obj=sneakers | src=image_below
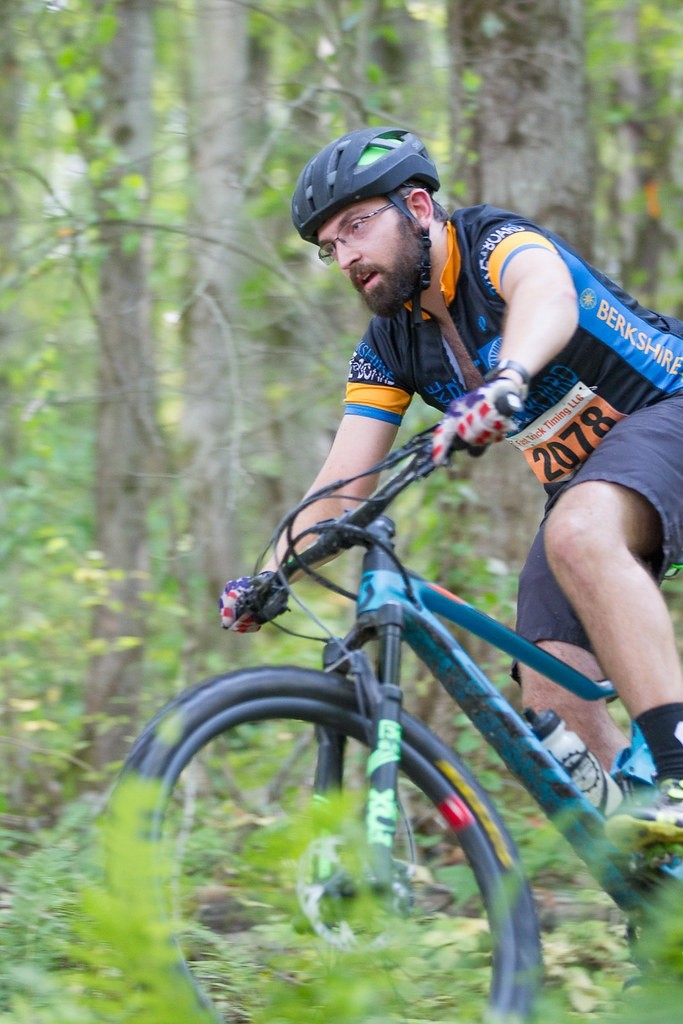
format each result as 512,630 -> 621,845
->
605,778 -> 683,854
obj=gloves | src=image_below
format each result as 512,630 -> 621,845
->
431,378 -> 521,464
218,571 -> 275,632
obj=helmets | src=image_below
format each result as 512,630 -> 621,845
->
291,126 -> 440,245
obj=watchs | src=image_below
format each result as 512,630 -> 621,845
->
485,359 -> 529,386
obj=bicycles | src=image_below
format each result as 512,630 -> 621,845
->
111,424 -> 682,1024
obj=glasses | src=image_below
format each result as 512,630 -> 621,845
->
318,187 -> 428,265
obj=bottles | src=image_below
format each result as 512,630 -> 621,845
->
522,707 -> 623,815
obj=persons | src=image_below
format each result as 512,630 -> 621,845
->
219,121 -> 683,887
